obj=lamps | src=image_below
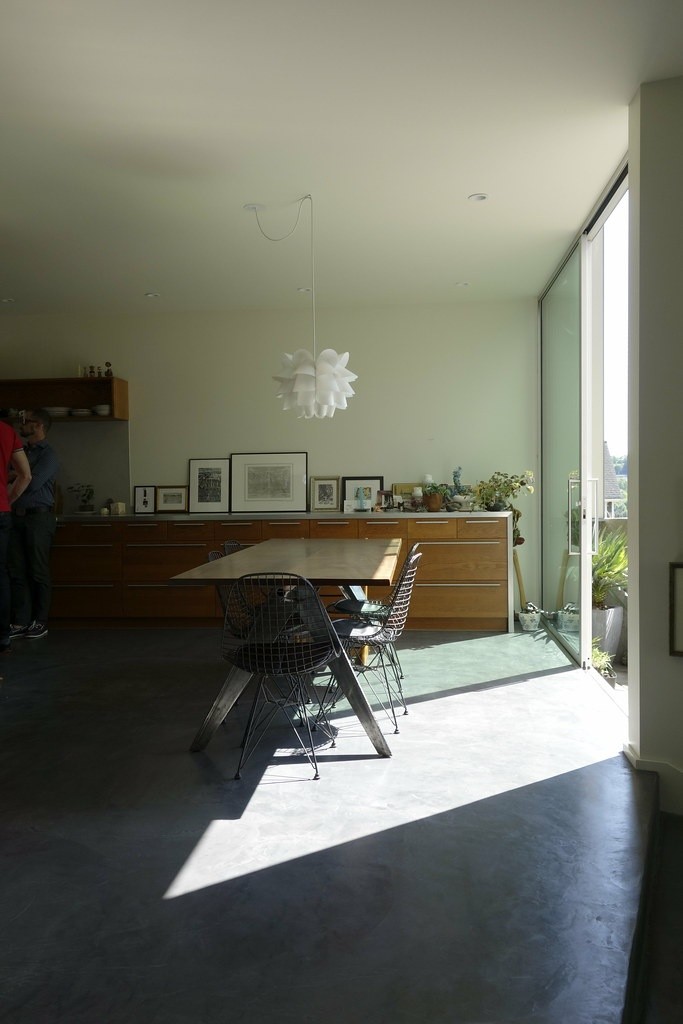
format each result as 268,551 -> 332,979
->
243,197 -> 359,419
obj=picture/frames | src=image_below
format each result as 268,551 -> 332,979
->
310,476 -> 341,512
188,458 -> 230,513
341,476 -> 384,511
155,485 -> 188,513
230,452 -> 308,512
134,486 -> 155,513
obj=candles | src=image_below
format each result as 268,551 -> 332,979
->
414,487 -> 423,496
424,474 -> 432,483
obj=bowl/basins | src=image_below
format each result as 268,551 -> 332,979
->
95,405 -> 111,416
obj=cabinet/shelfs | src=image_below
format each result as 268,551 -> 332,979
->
49,514 -> 514,633
0,377 -> 129,421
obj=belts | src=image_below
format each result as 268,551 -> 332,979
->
12,504 -> 53,517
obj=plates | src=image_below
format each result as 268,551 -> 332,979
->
40,407 -> 71,417
70,409 -> 93,416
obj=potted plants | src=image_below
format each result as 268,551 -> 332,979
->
558,602 -> 580,631
592,525 -> 628,666
423,465 -> 534,546
68,483 -> 95,512
592,636 -> 617,689
518,601 -> 541,631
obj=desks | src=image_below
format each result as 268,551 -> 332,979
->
167,538 -> 402,757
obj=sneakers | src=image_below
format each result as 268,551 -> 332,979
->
6,624 -> 30,639
25,620 -> 49,638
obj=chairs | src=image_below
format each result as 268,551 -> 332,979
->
203,539 -> 422,780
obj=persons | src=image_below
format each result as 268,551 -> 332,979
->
0,417 -> 32,651
23,410 -> 63,640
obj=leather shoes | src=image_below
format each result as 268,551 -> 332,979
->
0,637 -> 13,652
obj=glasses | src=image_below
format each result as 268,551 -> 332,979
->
21,419 -> 39,425
